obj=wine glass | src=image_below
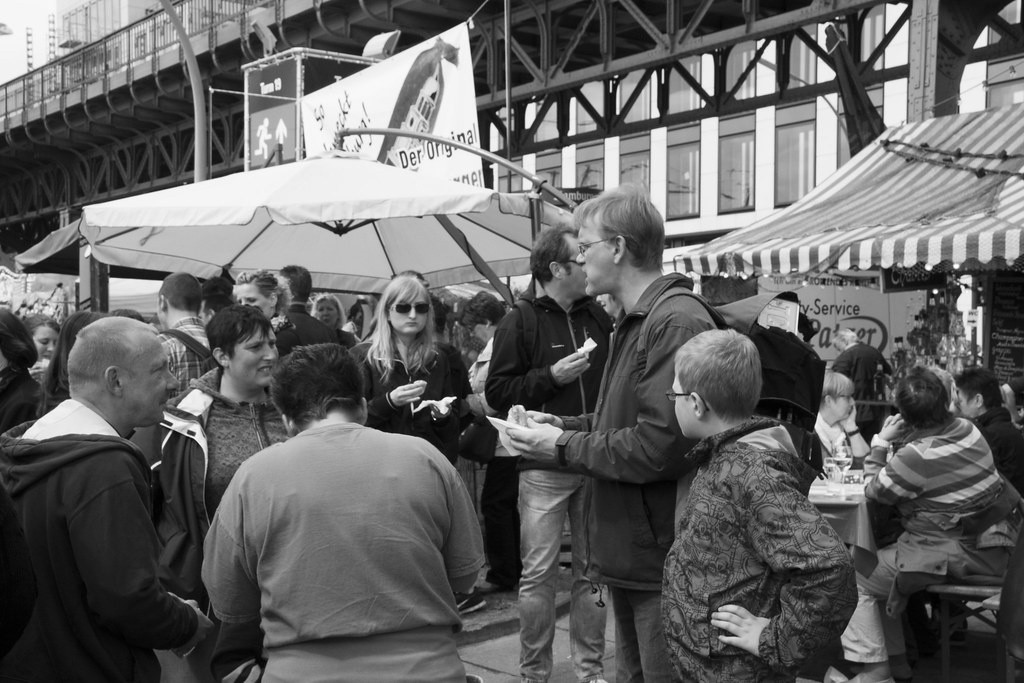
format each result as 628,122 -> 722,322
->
824,445 -> 854,494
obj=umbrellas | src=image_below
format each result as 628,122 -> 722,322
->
13,125 -> 589,299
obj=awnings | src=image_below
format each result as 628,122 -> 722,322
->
672,102 -> 1024,280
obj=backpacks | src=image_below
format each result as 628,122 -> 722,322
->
635,289 -> 823,470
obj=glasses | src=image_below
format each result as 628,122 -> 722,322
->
578,237 -> 612,256
390,303 -> 429,313
839,394 -> 851,399
467,324 -> 477,332
665,389 -> 710,412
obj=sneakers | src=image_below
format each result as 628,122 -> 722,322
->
453,586 -> 486,615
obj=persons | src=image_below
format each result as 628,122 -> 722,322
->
201,344 -> 489,681
0,264 -> 1023,683
658,328 -> 858,683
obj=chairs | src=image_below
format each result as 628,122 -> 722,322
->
923,583 -> 1024,683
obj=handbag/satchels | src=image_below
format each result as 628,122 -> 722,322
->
458,416 -> 499,466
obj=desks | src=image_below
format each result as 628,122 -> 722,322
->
809,469 -> 898,579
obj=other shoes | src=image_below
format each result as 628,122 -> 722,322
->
906,652 -> 919,666
847,664 -> 914,683
586,679 -> 609,683
929,614 -> 969,645
486,570 -> 513,592
915,645 -> 935,656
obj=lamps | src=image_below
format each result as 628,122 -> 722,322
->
361,29 -> 402,59
